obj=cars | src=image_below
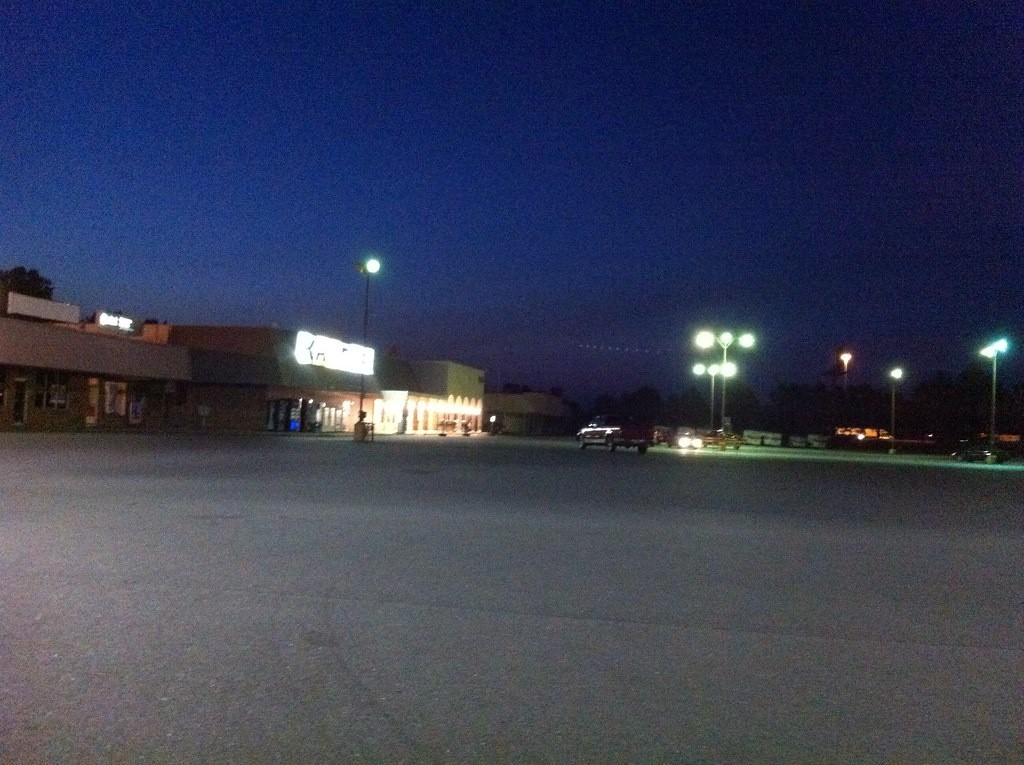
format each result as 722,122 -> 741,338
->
703,430 -> 744,449
666,427 -> 703,449
947,442 -> 1010,464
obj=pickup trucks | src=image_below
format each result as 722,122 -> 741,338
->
576,413 -> 655,454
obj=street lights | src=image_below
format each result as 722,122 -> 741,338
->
490,415 -> 496,432
888,368 -> 902,455
840,353 -> 852,384
980,338 -> 1008,442
359,259 -> 381,421
693,331 -> 755,430
